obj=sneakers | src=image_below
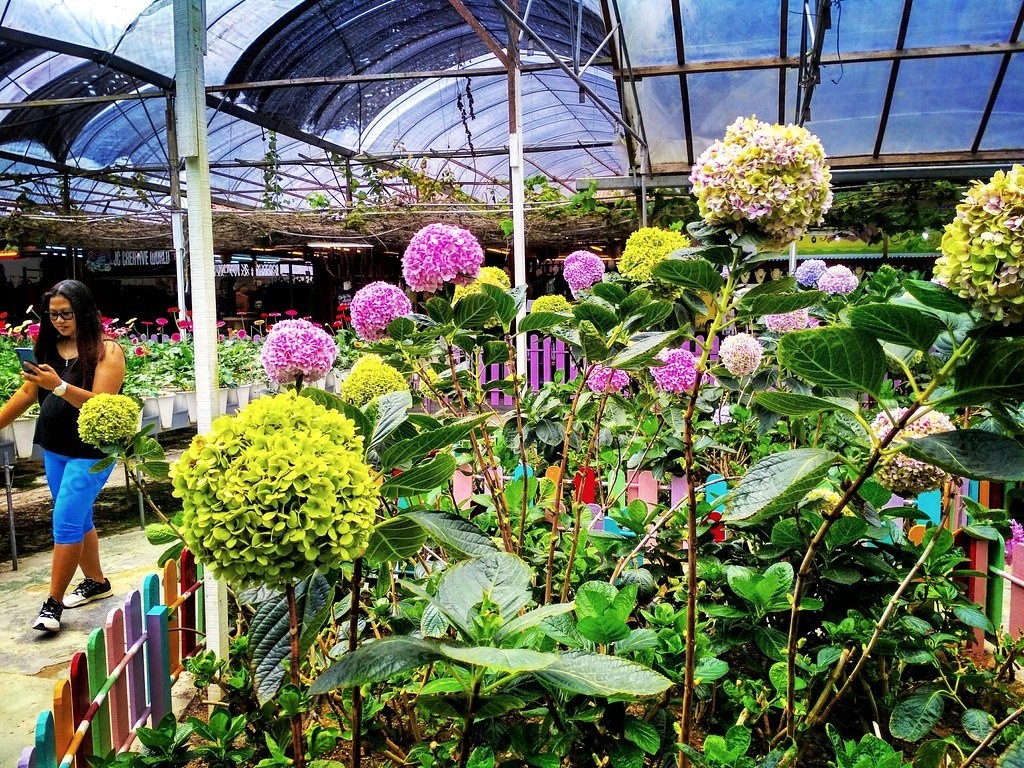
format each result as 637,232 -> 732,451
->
31,596 -> 66,632
62,577 -> 114,608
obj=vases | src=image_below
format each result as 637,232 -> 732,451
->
250,382 -> 271,399
327,368 -> 341,388
311,376 -> 326,390
0,418 -> 37,458
143,392 -> 176,428
185,389 -> 229,421
168,389 -> 188,415
0,303 -> 360,418
335,371 -> 350,393
228,383 -> 252,411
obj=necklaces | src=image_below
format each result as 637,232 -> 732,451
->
64,341 -> 77,366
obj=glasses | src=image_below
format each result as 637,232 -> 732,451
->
44,311 -> 74,321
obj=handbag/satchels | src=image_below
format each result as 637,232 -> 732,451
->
42,356 -> 94,424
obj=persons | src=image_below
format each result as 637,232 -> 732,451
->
0,280 -> 126,630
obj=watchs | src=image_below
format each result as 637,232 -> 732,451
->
52,380 -> 69,396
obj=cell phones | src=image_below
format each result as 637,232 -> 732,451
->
14,348 -> 39,375
